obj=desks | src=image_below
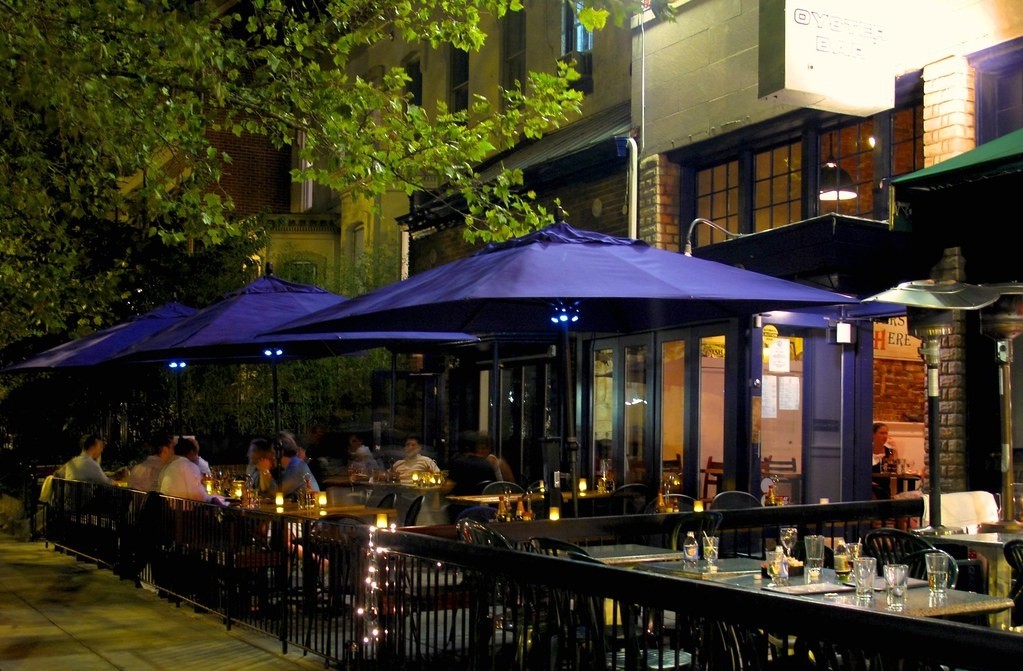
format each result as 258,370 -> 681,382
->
871,471 -> 920,480
922,531 -> 1023,635
222,475 -> 451,619
793,589 -> 1014,671
713,572 -> 930,597
445,492 -> 544,507
575,543 -> 683,566
560,489 -> 610,499
636,557 -> 772,580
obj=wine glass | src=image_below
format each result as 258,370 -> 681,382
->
780,528 -> 798,557
702,537 -> 719,570
766,551 -> 784,587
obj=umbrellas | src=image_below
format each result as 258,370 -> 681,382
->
82,262 -> 482,492
255,203 -> 864,516
0,300 -> 289,439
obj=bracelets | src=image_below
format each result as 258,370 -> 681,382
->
263,470 -> 270,475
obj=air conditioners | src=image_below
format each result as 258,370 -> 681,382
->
556,49 -> 591,79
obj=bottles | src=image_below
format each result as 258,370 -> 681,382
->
495,496 -> 533,522
764,485 -> 789,506
654,489 -> 680,513
883,457 -> 915,475
296,485 -> 319,511
683,531 -> 699,571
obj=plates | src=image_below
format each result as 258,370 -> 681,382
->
760,583 -> 856,595
843,576 -> 929,591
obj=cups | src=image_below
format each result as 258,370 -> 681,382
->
883,564 -> 909,612
834,540 -> 863,584
925,553 -> 949,593
804,535 -> 825,568
853,557 -> 877,601
411,469 -> 444,487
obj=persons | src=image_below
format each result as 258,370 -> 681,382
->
381,432 -> 441,478
872,422 -> 900,473
245,430 -> 320,539
306,425 -> 372,466
54,434 -> 120,512
440,432 -> 515,513
127,435 -> 213,543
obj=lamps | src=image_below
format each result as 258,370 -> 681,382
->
818,131 -> 858,200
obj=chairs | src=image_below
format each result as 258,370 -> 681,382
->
405,494 -> 424,528
452,455 -> 1023,671
376,492 -> 398,508
290,514 -> 369,631
